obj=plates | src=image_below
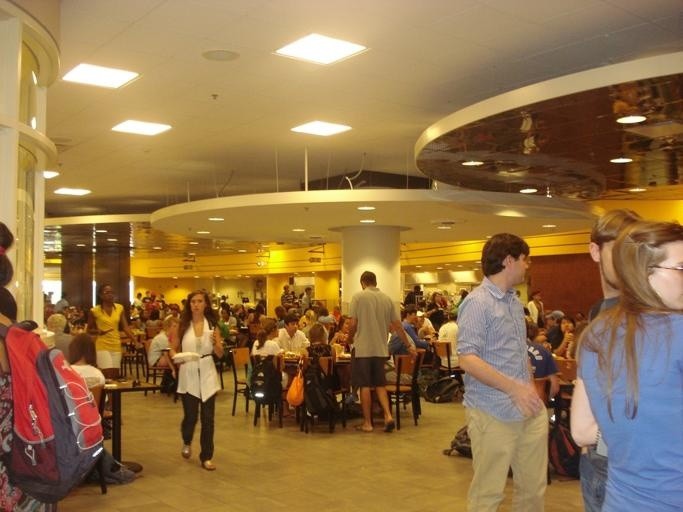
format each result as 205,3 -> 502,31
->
283,345 -> 352,362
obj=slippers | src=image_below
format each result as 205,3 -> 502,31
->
354,424 -> 373,432
384,418 -> 395,432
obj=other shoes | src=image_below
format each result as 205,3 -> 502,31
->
182,444 -> 192,460
203,461 -> 216,471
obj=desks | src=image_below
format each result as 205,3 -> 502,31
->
215,344 -> 237,389
104,377 -> 162,475
281,352 -> 354,427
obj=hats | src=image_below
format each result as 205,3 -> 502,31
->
545,310 -> 565,320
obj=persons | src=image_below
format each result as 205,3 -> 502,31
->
387,283 -> 470,385
516,288 -> 587,398
455,232 -> 549,512
344,271 -> 412,433
0,312 -> 58,512
44,283 -> 186,408
216,284 -> 351,421
579,208 -> 642,512
168,290 -> 225,471
570,220 -> 682,512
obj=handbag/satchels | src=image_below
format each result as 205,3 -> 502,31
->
285,358 -> 306,407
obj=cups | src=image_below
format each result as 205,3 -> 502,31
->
207,329 -> 214,342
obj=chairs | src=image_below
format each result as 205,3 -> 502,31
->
249,355 -> 287,427
247,323 -> 259,348
229,347 -> 251,416
373,353 -> 422,431
120,334 -> 146,379
433,342 -> 465,389
142,339 -> 171,395
535,377 -> 552,408
415,348 -> 426,385
327,363 -> 352,427
296,358 -> 335,433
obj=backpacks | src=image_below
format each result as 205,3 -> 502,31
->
544,423 -> 577,480
304,356 -> 345,425
251,356 -> 281,407
443,423 -> 472,459
4,320 -> 104,501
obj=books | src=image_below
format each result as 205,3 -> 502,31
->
594,431 -> 609,458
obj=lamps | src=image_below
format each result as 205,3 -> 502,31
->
255,241 -> 270,268
308,239 -> 326,263
182,245 -> 196,270
